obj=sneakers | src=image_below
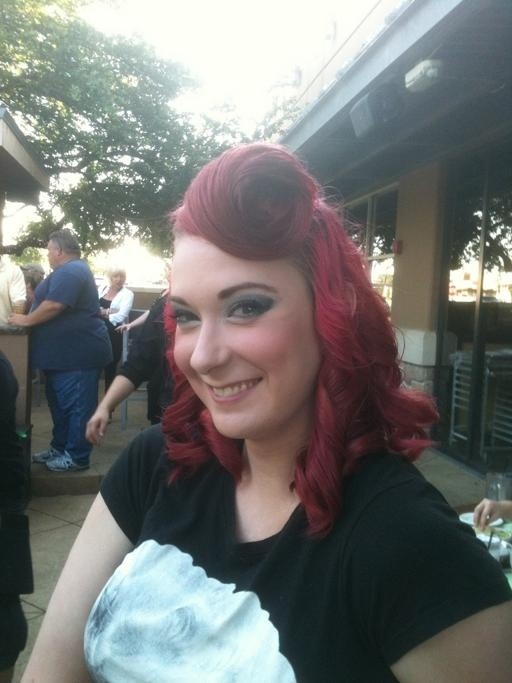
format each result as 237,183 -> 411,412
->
109,412 -> 113,424
32,447 -> 89,472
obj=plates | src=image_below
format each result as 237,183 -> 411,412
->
458,510 -> 504,528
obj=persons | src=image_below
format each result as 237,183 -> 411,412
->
0,228 -> 174,471
472,497 -> 512,531
23,142 -> 512,683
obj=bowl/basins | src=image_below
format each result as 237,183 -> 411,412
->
476,535 -> 500,549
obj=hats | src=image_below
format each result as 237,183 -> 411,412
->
20,262 -> 45,274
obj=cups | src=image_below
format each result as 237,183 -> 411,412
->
485,471 -> 506,501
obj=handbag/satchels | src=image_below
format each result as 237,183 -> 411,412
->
10,513 -> 35,596
